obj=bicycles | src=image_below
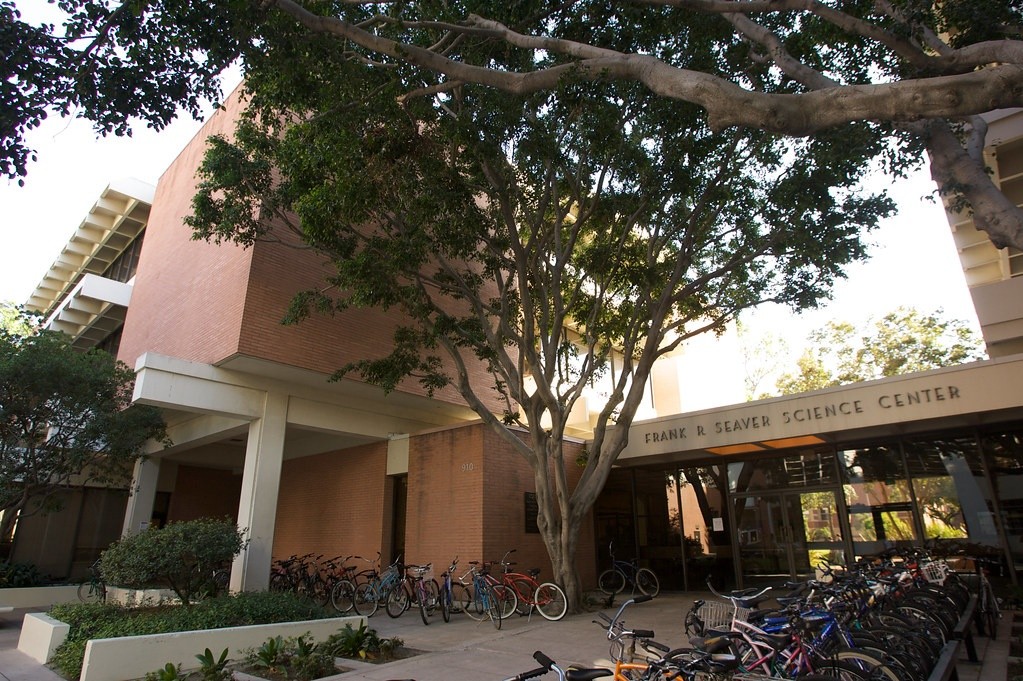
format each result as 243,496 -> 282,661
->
268,535 -> 1007,681
597,541 -> 661,601
77,558 -> 107,606
